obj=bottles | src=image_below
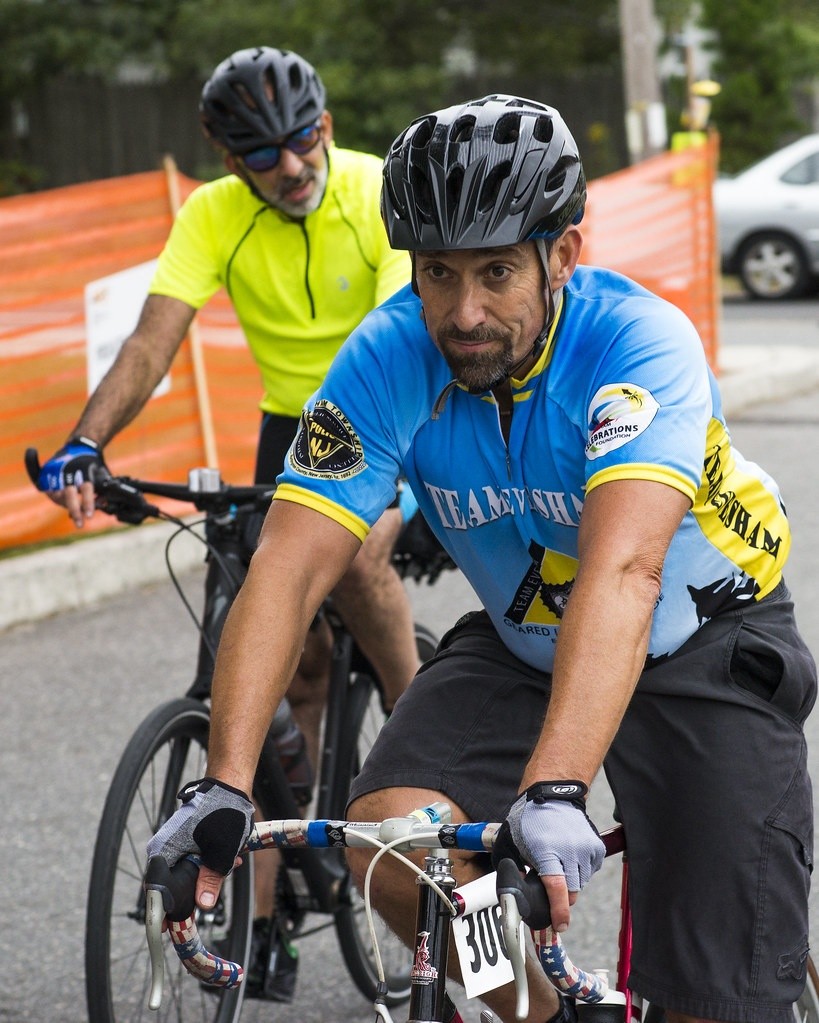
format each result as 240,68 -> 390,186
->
271,697 -> 314,805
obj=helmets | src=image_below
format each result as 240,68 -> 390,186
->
381,94 -> 586,252
199,45 -> 325,152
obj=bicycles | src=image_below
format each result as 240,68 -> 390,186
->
143,803 -> 819,1023
24,446 -> 442,1023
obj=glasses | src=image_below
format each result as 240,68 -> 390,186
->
238,120 -> 321,173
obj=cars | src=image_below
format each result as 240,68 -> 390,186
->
711,131 -> 819,301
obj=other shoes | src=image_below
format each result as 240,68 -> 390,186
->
201,937 -> 298,1002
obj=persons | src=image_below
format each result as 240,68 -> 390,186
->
145,94 -> 819,1023
37,46 -> 423,996
